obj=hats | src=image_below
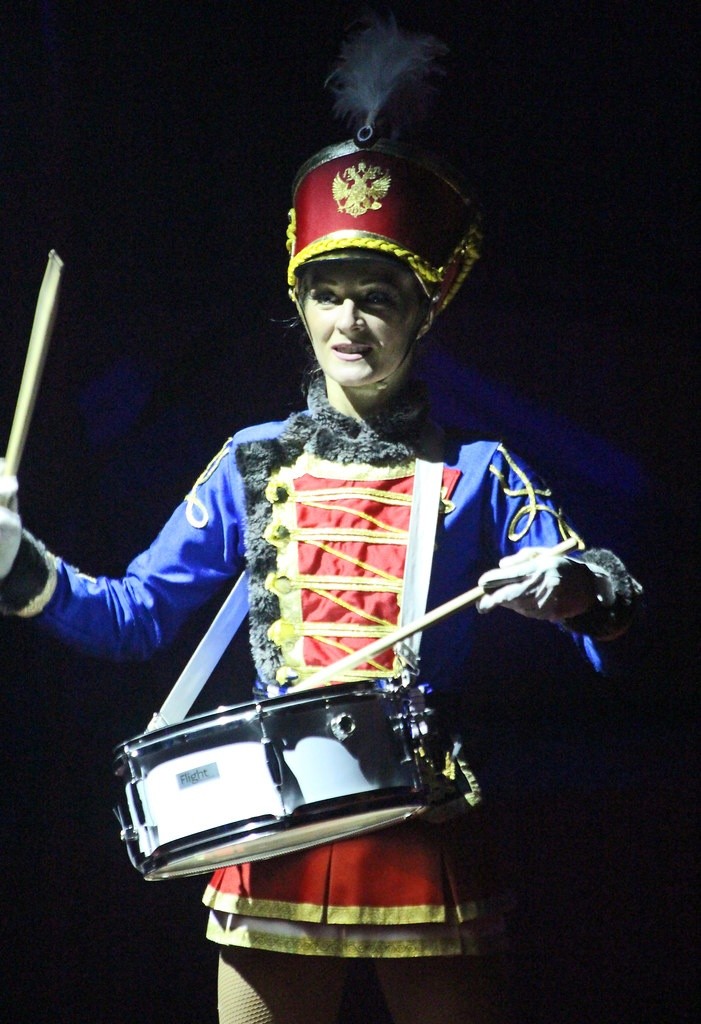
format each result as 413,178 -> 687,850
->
288,140 -> 485,316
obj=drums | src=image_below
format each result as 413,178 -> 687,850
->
104,673 -> 442,889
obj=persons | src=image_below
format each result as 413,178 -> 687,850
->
0,137 -> 672,1023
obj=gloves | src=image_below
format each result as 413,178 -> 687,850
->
475,546 -> 589,620
0,457 -> 22,580
